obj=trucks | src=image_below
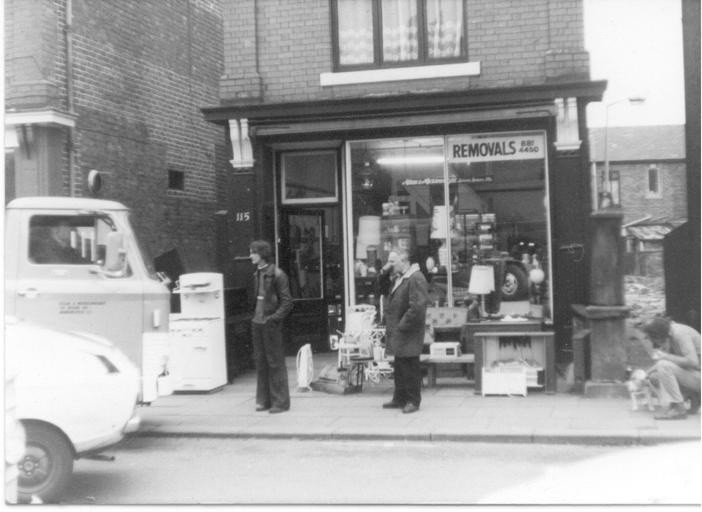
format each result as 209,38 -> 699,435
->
452,226 -> 527,299
5,173 -> 175,429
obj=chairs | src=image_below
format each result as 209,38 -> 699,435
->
337,304 -> 376,370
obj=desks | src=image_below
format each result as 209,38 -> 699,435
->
463,314 -> 543,380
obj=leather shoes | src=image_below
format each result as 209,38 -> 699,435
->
382,400 -> 419,414
256,403 -> 288,414
654,408 -> 687,421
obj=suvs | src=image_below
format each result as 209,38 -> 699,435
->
6,315 -> 146,505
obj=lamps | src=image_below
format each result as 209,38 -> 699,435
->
358,215 -> 381,267
429,205 -> 457,267
467,264 -> 501,318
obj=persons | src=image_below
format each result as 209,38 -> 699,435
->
246,241 -> 293,414
382,248 -> 428,413
31,216 -> 93,264
642,316 -> 701,419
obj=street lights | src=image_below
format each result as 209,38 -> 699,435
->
599,94 -> 648,195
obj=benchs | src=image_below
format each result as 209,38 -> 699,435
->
387,353 -> 476,388
